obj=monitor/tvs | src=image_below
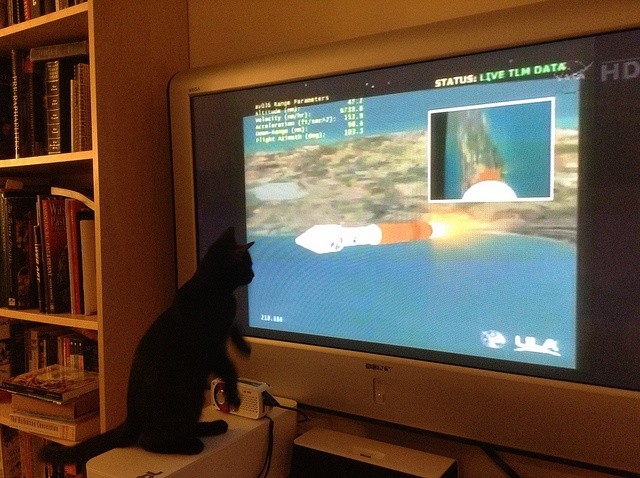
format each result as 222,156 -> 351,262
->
167,0 -> 640,477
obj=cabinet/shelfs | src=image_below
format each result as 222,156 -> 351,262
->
0,1 -> 188,478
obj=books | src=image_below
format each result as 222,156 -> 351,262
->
0,177 -> 97,317
11,41 -> 92,159
0,1 -> 87,28
0,323 -> 101,441
0,442 -> 87,478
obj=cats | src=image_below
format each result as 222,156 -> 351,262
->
38,225 -> 255,466
